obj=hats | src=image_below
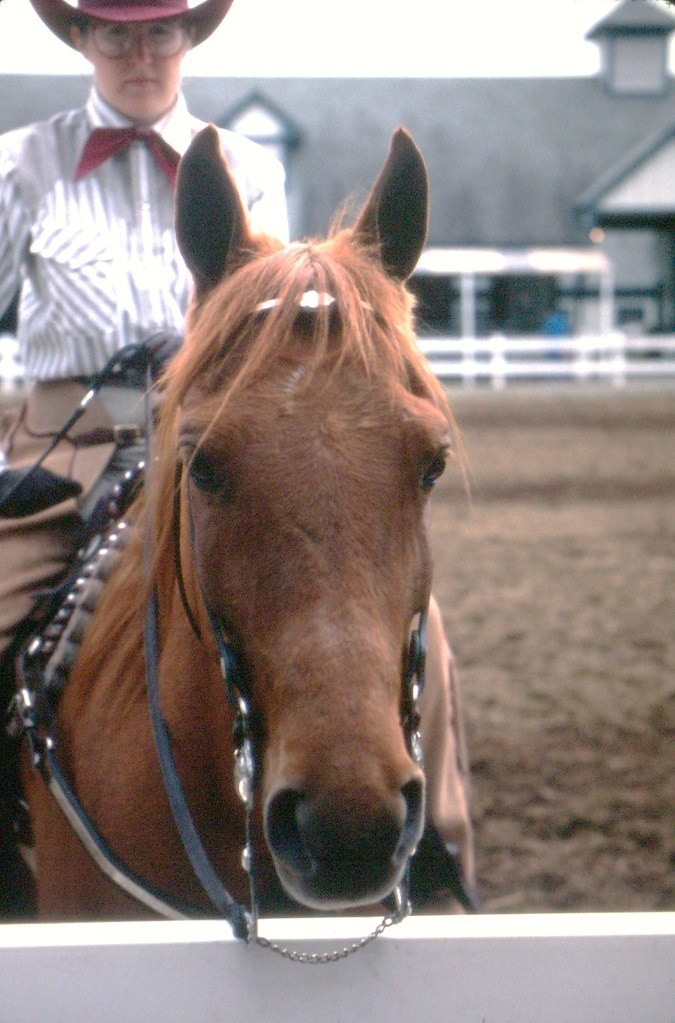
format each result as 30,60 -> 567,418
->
33,0 -> 233,53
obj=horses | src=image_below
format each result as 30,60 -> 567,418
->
26,122 -> 478,922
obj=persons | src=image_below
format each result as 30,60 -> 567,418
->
0,0 -> 474,916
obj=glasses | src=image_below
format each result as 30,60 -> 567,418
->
82,19 -> 188,59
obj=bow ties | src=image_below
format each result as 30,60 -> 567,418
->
73,126 -> 180,184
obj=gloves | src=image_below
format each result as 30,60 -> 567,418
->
0,465 -> 82,516
121,333 -> 184,374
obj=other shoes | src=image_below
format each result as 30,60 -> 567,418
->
415,892 -> 464,915
